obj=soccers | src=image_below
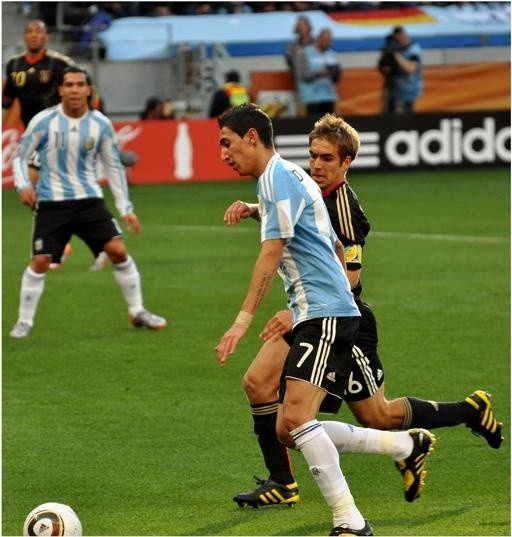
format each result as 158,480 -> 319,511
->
24,502 -> 81,536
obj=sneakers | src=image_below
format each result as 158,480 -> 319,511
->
232,474 -> 300,509
89,252 -> 109,272
9,321 -> 33,339
465,390 -> 503,449
395,429 -> 437,502
329,519 -> 375,537
48,244 -> 71,270
127,308 -> 167,331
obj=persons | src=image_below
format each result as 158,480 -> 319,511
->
207,70 -> 254,118
213,103 -> 436,534
139,97 -> 175,120
294,30 -> 343,132
379,25 -> 424,111
223,113 -> 504,508
3,20 -> 138,170
285,15 -> 322,70
8,67 -> 167,338
